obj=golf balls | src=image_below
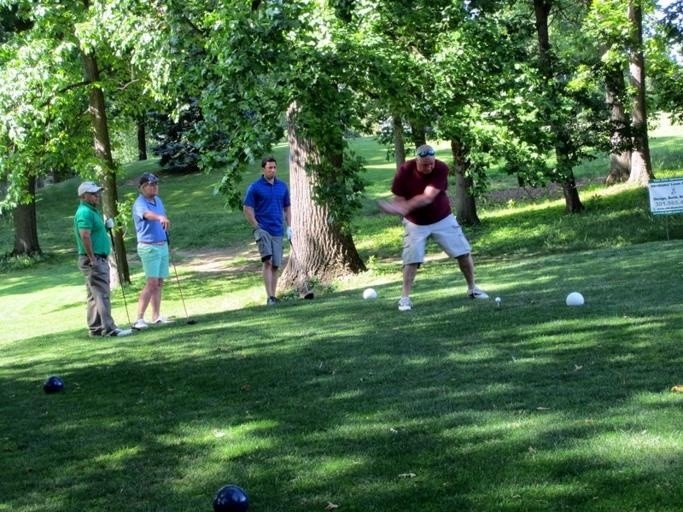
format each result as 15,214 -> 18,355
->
362,288 -> 377,301
495,298 -> 502,304
566,292 -> 584,307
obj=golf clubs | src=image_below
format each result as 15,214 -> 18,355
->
288,238 -> 313,297
109,225 -> 138,329
164,228 -> 189,320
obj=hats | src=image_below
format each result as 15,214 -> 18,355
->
140,173 -> 159,184
78,182 -> 104,196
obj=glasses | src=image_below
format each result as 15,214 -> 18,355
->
418,150 -> 434,157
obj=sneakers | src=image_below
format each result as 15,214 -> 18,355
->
88,319 -> 173,337
268,296 -> 276,305
468,287 -> 489,299
397,297 -> 412,311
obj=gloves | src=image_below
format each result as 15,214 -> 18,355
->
105,218 -> 114,228
286,226 -> 291,239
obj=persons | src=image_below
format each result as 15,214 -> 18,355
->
129,172 -> 175,330
71,182 -> 132,337
376,144 -> 489,311
243,156 -> 292,306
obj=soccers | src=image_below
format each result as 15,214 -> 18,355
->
43,376 -> 65,394
212,485 -> 248,511
